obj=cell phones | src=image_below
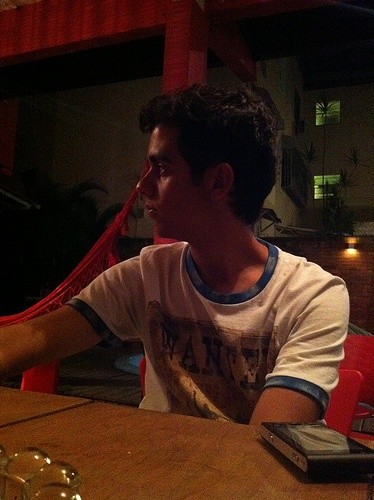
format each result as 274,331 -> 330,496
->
260,420 -> 374,477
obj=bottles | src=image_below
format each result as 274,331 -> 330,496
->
4,448 -> 49,500
27,461 -> 82,500
0,445 -> 11,473
1,473 -> 28,500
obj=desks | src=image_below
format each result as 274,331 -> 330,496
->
0,387 -> 374,499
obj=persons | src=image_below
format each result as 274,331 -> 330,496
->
0,83 -> 351,427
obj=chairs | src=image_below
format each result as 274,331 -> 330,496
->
138,332 -> 374,441
